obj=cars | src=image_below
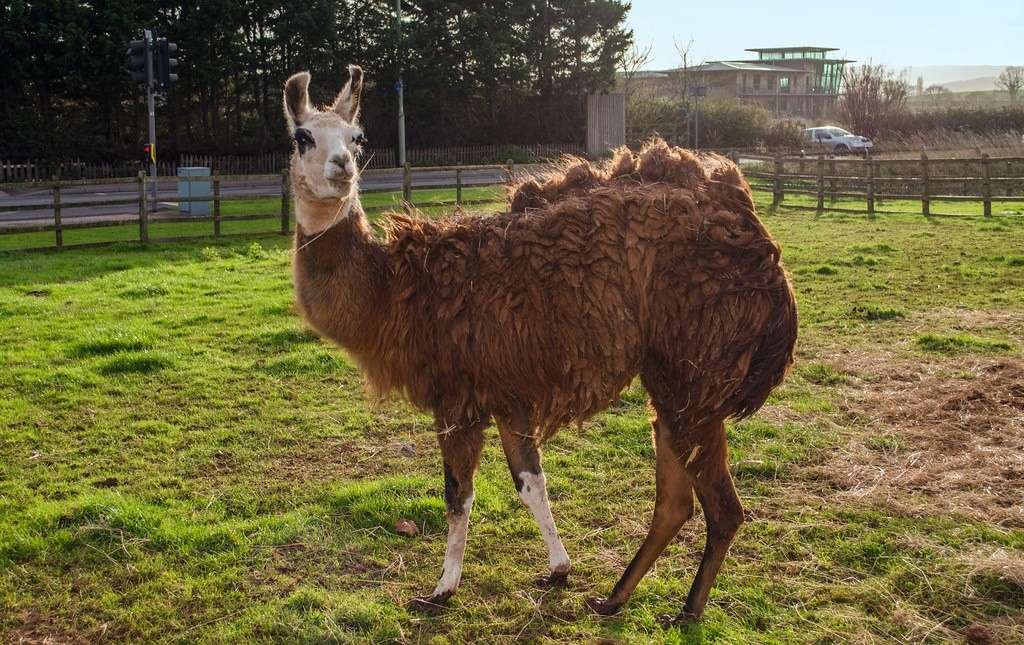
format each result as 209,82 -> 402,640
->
799,126 -> 874,153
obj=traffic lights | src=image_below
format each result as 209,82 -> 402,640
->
128,30 -> 150,85
163,37 -> 180,87
144,143 -> 153,162
